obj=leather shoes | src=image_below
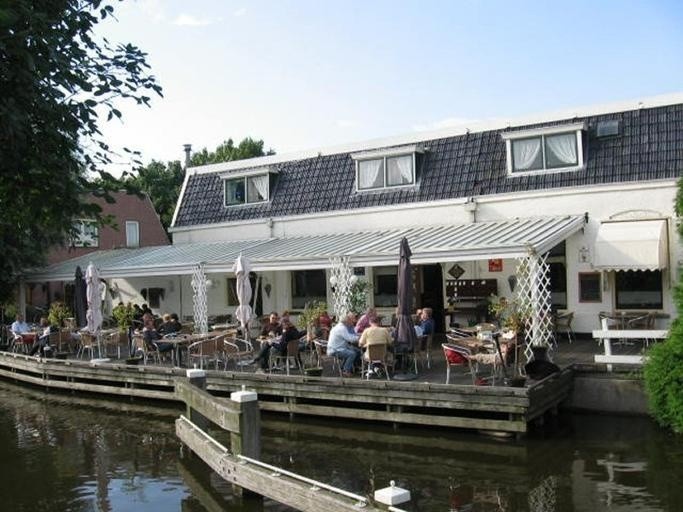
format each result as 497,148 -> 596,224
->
343,371 -> 352,377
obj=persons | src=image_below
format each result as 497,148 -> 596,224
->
29,318 -> 57,356
297,317 -> 321,365
10,313 -> 31,342
158,314 -> 180,333
318,310 -> 331,326
325,305 -> 435,378
281,310 -> 290,325
260,311 -> 280,338
247,318 -> 303,373
142,312 -> 186,367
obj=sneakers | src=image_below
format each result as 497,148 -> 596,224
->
365,367 -> 384,378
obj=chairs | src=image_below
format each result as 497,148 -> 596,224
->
598,308 -> 657,349
9,322 -> 522,385
552,310 -> 575,346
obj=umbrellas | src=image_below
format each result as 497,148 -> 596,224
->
395,236 -> 416,355
233,252 -> 258,352
81,260 -> 101,337
72,265 -> 87,328
